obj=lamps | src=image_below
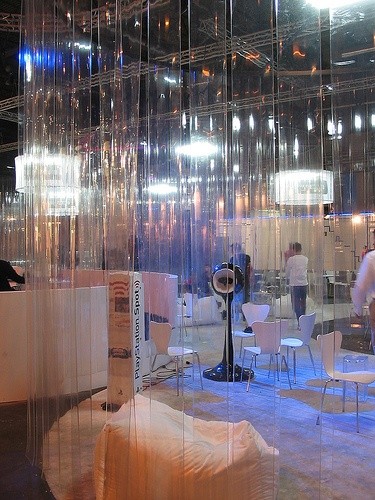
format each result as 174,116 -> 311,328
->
273,169 -> 334,206
33,188 -> 80,216
14,154 -> 81,195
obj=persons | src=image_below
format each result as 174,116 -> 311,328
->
284,242 -> 309,331
0,259 -> 26,292
230,242 -> 252,333
184,263 -> 214,298
352,230 -> 375,356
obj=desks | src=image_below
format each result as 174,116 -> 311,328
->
9,277 -> 72,289
273,276 -> 291,279
322,274 -> 361,276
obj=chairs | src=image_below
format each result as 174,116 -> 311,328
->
149,270 -> 375,433
